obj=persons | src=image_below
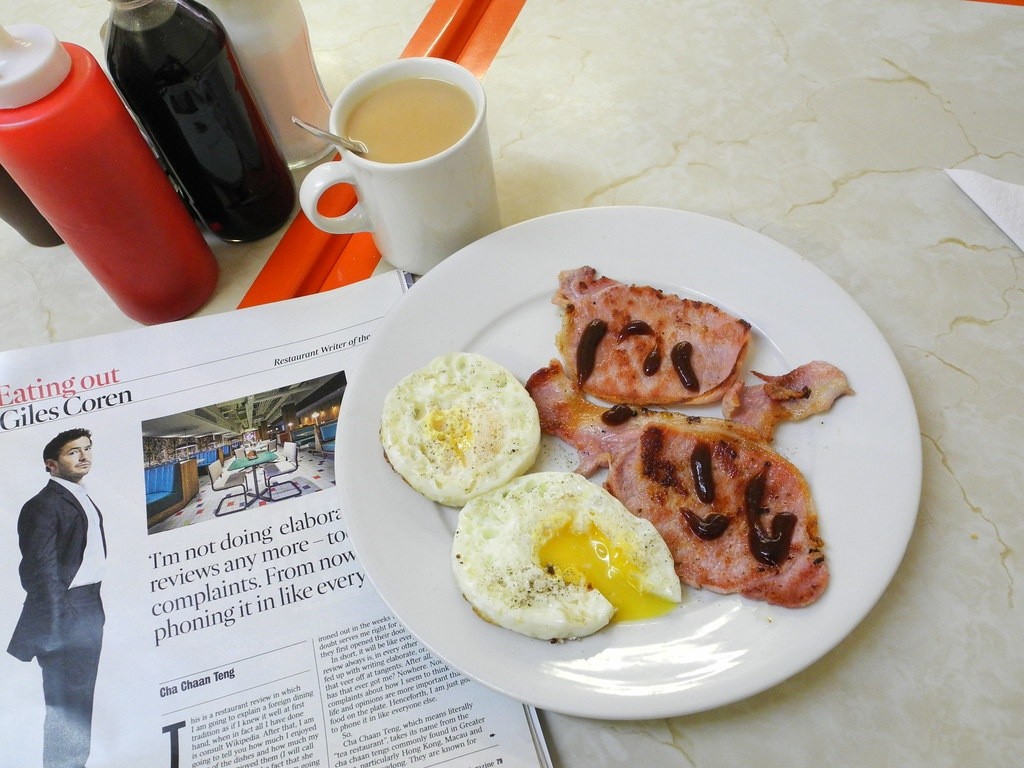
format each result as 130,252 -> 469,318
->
5,427 -> 109,767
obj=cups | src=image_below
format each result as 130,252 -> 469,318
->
298,57 -> 508,276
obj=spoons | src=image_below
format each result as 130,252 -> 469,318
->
289,114 -> 367,156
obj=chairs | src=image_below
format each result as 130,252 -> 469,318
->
145,420 -> 338,530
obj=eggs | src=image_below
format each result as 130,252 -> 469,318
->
388,353 -> 681,642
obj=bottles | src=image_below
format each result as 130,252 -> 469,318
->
100,0 -> 295,245
196,0 -> 341,170
2,22 -> 220,328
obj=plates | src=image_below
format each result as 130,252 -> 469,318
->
330,206 -> 925,723
